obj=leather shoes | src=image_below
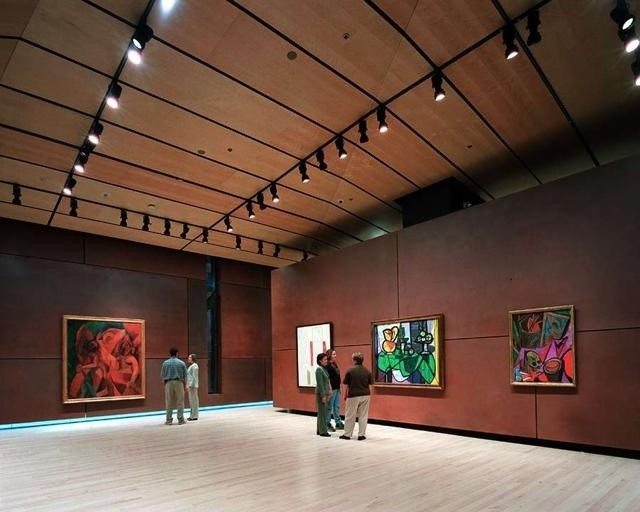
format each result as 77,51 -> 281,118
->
320,433 -> 331,437
358,436 -> 366,440
339,435 -> 350,440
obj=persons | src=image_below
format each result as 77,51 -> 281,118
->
160,347 -> 189,425
186,354 -> 199,421
315,353 -> 331,436
339,352 -> 374,440
325,348 -> 344,432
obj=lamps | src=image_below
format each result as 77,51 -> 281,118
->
604,1 -> 640,89
60,0 -> 184,200
6,184 -> 318,265
222,1 -> 551,234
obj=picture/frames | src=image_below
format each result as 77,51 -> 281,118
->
62,314 -> 146,404
507,304 -> 577,387
371,311 -> 446,392
295,322 -> 333,388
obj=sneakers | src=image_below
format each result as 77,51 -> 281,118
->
335,422 -> 345,430
326,423 -> 336,433
165,417 -> 198,425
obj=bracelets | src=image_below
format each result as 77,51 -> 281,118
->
185,385 -> 188,387
